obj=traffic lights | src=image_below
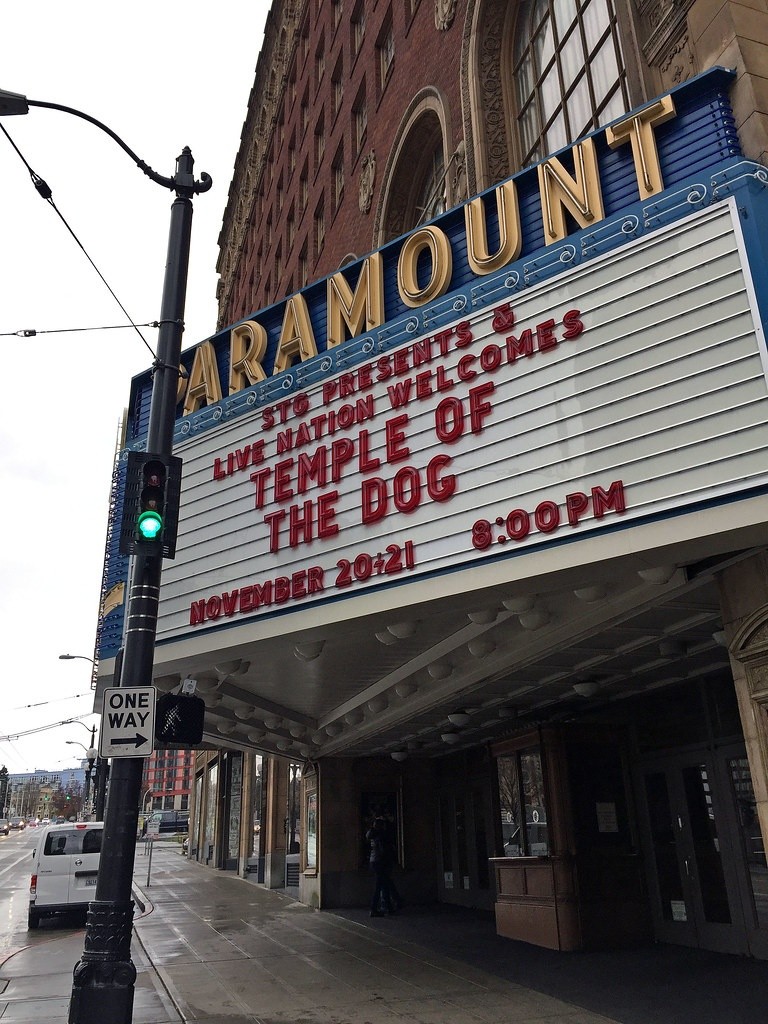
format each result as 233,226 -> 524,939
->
137,459 -> 170,546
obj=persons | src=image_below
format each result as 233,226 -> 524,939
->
365,809 -> 401,919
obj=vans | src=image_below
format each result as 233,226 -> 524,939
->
142,812 -> 188,833
27,821 -> 104,926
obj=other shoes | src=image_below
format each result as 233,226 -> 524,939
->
369,897 -> 399,917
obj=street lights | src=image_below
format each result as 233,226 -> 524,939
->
142,788 -> 173,813
0,87 -> 212,1024
59,655 -> 111,821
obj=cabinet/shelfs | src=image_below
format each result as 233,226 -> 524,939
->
494,862 -> 560,951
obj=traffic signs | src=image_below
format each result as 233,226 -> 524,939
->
99,685 -> 158,759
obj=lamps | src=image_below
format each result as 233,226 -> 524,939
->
441,734 -> 460,745
391,752 -> 408,762
572,681 -> 600,698
448,714 -> 470,727
153,566 -> 678,758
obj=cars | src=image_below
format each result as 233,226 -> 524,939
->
0,819 -> 10,836
28,816 -> 76,827
254,819 -> 259,836
8,817 -> 26,830
